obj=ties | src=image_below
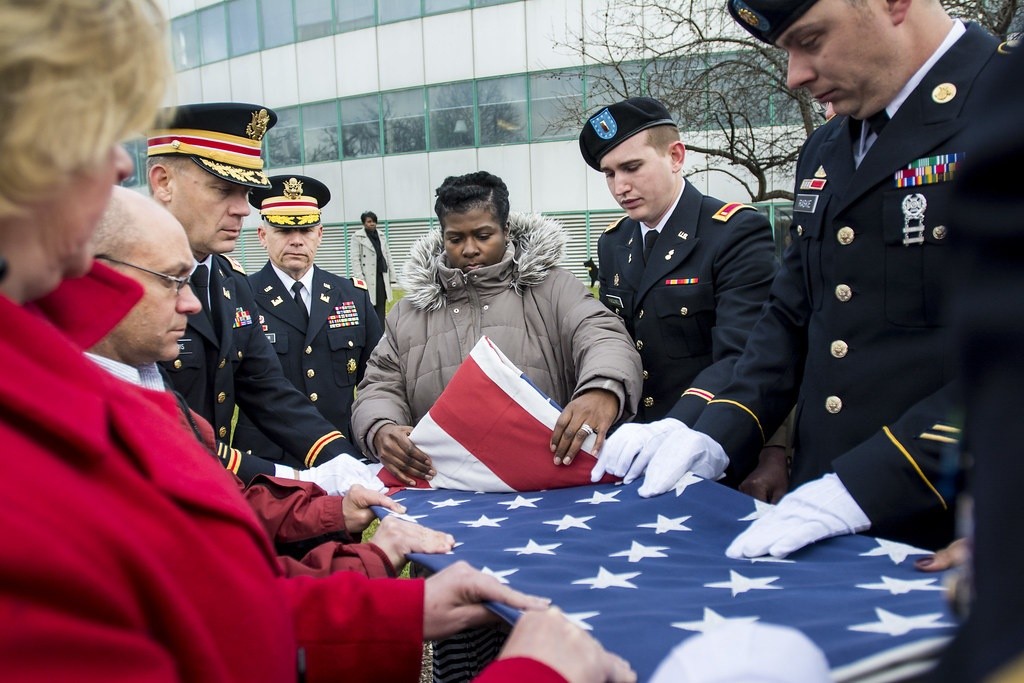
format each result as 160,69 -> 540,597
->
292,282 -> 309,325
643,230 -> 660,266
867,107 -> 891,136
191,265 -> 213,322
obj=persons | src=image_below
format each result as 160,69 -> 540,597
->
577,97 -> 781,482
79,184 -> 455,578
349,212 -> 395,331
144,102 -> 386,497
1,0 -> 639,683
350,171 -> 643,487
231,174 -> 386,471
638,0 -> 1024,560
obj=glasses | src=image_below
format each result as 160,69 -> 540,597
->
93,255 -> 192,296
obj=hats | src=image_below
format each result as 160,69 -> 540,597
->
147,103 -> 278,189
727,0 -> 818,44
579,97 -> 679,172
248,175 -> 331,228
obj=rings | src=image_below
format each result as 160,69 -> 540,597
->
581,424 -> 593,435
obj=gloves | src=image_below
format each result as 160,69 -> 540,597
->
647,620 -> 834,683
299,453 -> 389,498
725,473 -> 871,559
638,428 -> 730,498
591,418 -> 690,484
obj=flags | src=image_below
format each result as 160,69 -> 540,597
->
365,334 -> 969,683
